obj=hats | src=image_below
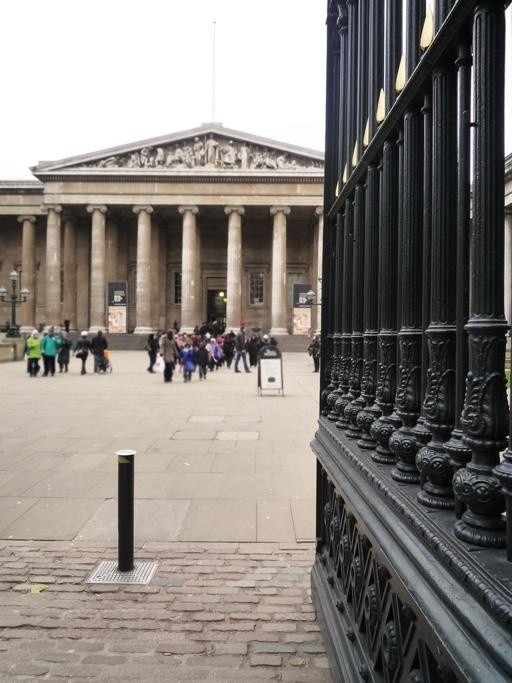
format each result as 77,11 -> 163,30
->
49,328 -> 54,332
33,330 -> 38,335
81,330 -> 88,336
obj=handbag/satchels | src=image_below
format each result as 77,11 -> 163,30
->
184,361 -> 195,370
76,353 -> 83,358
144,343 -> 152,350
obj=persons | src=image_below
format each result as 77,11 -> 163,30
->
19,328 -> 108,378
82,132 -> 307,173
308,335 -> 321,373
147,320 -> 278,383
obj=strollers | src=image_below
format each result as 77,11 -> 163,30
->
90,351 -> 113,375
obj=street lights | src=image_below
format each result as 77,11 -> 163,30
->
0,270 -> 33,338
